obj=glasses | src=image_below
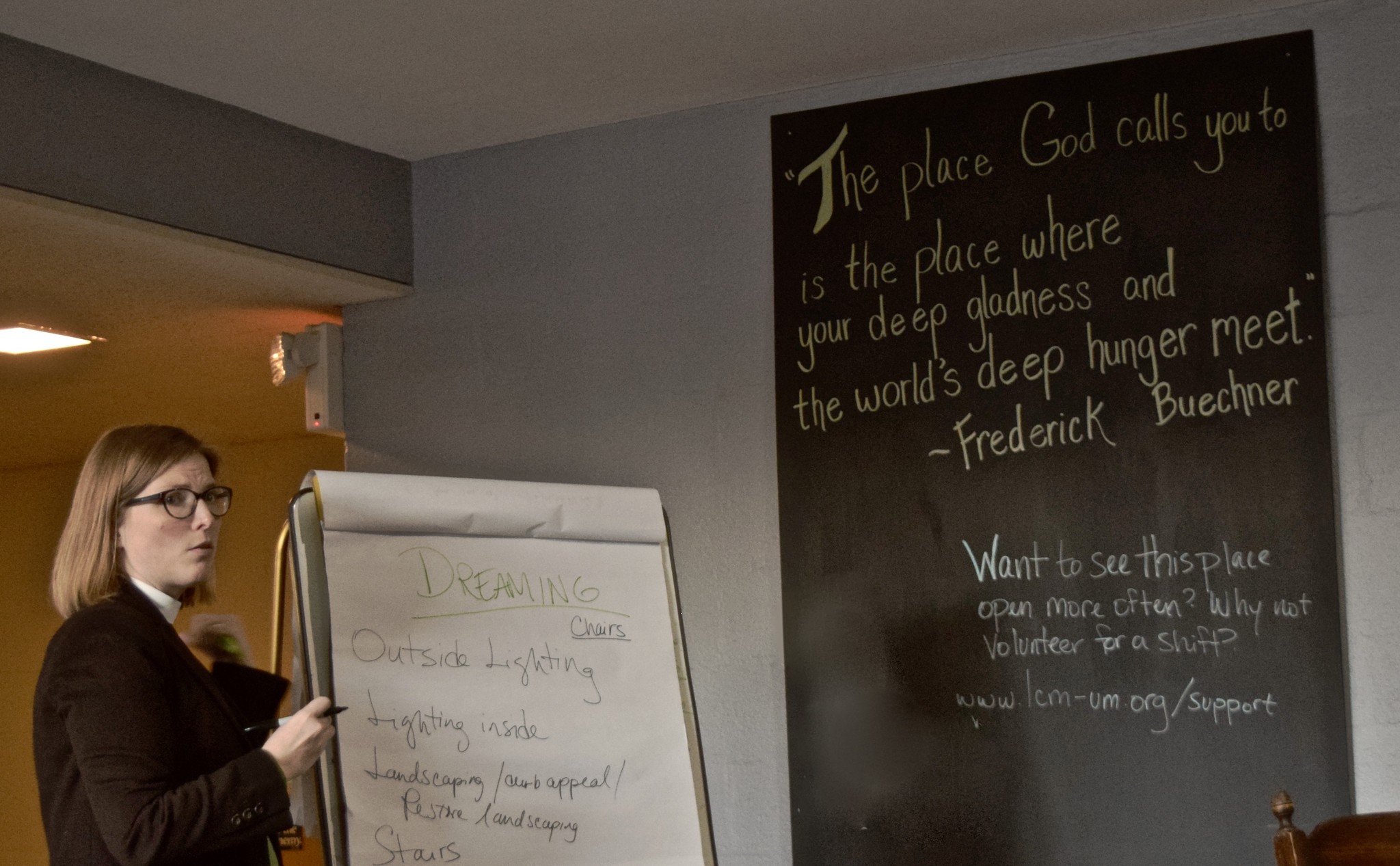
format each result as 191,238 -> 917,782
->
121,485 -> 233,520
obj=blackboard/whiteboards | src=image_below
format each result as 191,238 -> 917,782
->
769,28 -> 1358,866
293,484 -> 719,866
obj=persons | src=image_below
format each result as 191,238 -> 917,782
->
32,423 -> 336,866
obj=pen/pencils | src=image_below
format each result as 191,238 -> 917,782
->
243,706 -> 348,735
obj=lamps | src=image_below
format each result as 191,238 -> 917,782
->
271,321 -> 343,438
